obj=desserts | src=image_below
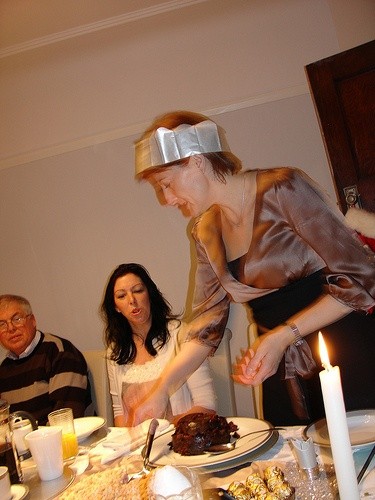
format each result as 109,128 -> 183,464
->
228,465 -> 293,500
168,412 -> 237,456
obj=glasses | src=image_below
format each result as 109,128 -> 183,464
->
0,314 -> 32,330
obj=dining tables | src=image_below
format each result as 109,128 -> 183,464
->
23,427 -> 375,500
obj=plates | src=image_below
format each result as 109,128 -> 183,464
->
10,483 -> 30,500
142,417 -> 280,475
301,408 -> 375,449
74,417 -> 109,442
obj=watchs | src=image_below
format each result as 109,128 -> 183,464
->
288,319 -> 305,348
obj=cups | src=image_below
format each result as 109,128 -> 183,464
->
48,407 -> 81,465
146,466 -> 204,500
24,427 -> 65,482
0,465 -> 12,500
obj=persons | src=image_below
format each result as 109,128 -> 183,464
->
0,294 -> 99,424
132,112 -> 375,425
101,263 -> 215,427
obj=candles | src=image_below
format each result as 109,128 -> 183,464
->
316,331 -> 359,500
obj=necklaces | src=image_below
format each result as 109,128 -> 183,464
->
222,173 -> 247,223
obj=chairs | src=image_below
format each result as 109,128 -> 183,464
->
207,327 -> 238,417
248,324 -> 263,420
82,351 -> 108,426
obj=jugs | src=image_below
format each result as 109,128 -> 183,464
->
0,399 -> 38,484
